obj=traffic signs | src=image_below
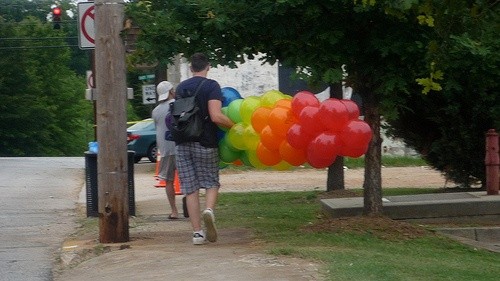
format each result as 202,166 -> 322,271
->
142,85 -> 157,105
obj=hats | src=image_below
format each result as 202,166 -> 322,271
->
158,82 -> 173,101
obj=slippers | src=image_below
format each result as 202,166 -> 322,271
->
167,213 -> 179,219
183,195 -> 189,218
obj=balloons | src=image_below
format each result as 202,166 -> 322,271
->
219,87 -> 372,169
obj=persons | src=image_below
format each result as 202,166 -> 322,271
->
175,52 -> 236,245
151,81 -> 189,220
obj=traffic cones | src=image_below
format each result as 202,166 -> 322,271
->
153,147 -> 167,187
173,168 -> 183,194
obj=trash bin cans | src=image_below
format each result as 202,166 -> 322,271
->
85,150 -> 137,216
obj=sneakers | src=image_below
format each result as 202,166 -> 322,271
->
204,211 -> 218,243
193,232 -> 205,245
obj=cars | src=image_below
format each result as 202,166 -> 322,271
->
126,118 -> 157,164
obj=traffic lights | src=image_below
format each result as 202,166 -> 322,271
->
52,7 -> 61,30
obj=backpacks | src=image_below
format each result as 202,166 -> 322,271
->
169,79 -> 214,141
